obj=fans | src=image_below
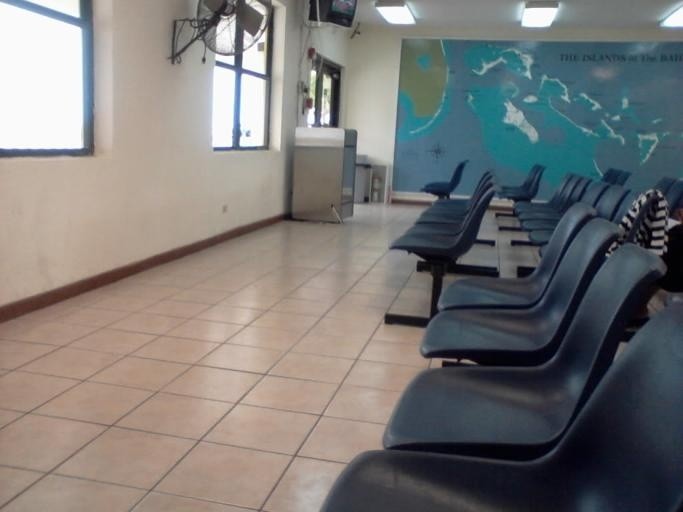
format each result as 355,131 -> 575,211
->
170,1 -> 273,65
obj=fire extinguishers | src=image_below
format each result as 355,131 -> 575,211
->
372,171 -> 382,202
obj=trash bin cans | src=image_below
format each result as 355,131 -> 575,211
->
353,154 -> 372,203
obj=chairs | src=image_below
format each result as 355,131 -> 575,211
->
418,217 -> 624,367
499,164 -> 681,345
386,156 -> 498,326
319,300 -> 681,511
380,241 -> 667,461
435,200 -> 598,314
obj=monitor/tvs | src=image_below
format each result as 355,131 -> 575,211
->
305,0 -> 358,28
